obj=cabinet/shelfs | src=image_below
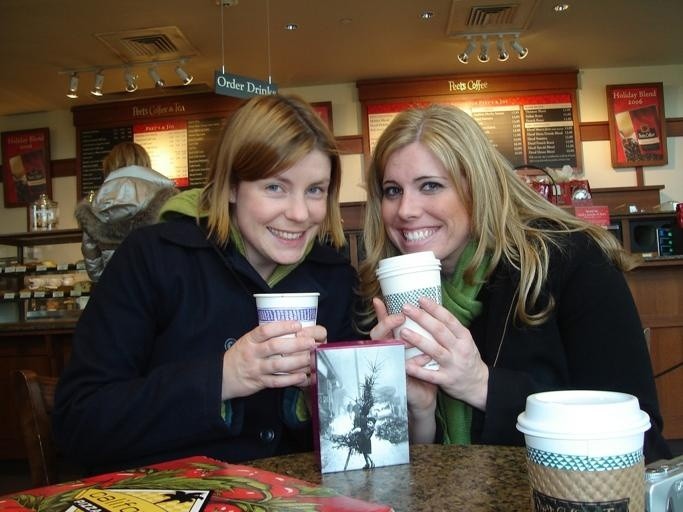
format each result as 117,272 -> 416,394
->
0,232 -> 92,329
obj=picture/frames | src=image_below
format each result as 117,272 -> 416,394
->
605,82 -> 667,168
0,128 -> 52,208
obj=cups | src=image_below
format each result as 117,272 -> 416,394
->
374,250 -> 444,373
253,290 -> 322,377
514,389 -> 654,510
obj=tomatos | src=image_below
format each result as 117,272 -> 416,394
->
0,456 -> 393,511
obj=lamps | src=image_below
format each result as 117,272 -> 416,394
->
451,31 -> 529,64
56,55 -> 195,99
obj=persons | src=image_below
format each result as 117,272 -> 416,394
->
70,141 -> 184,288
39,94 -> 363,483
346,98 -> 672,466
348,417 -> 378,468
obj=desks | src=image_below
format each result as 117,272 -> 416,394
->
0,443 -> 536,510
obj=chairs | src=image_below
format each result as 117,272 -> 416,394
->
11,368 -> 63,488
